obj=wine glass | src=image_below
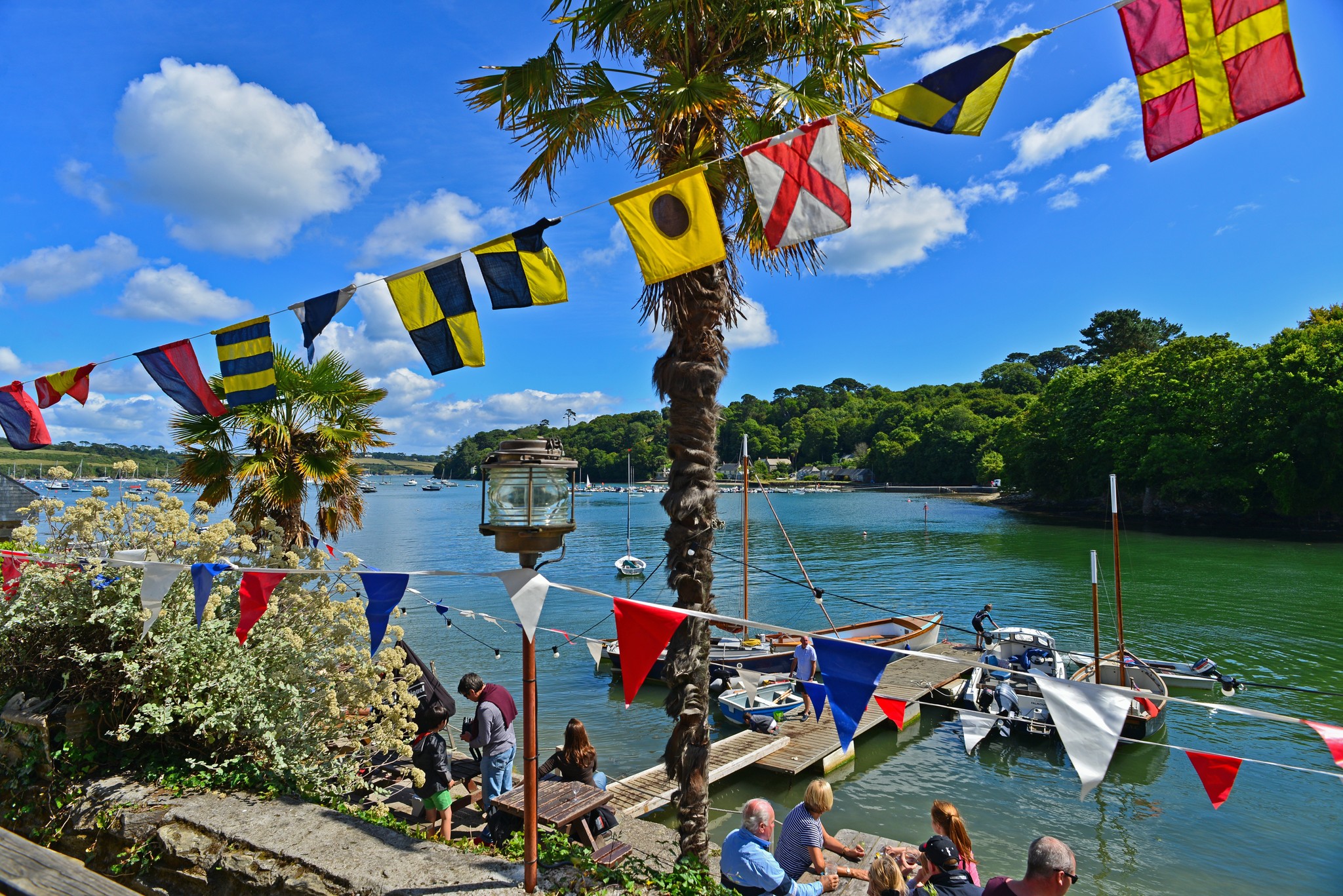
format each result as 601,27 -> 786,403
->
572,781 -> 581,802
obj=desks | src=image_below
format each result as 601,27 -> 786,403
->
490,772 -> 614,851
797,828 -> 921,896
319,716 -> 481,788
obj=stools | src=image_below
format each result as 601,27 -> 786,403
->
601,804 -> 616,816
588,841 -> 633,869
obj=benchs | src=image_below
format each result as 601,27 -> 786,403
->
1157,667 -> 1176,675
1100,683 -> 1162,695
340,773 -> 522,832
851,634 -> 898,643
775,691 -> 803,701
747,696 -> 778,706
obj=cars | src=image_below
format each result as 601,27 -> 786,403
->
568,474 -> 669,493
719,486 -> 839,494
789,473 -> 795,477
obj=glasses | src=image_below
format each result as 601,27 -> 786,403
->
463,690 -> 471,698
1053,868 -> 1078,884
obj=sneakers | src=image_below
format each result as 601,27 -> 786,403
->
798,708 -> 811,715
801,713 -> 809,722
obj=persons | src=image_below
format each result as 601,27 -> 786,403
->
719,780 -> 1078,896
972,604 -> 1000,647
458,673 -> 517,848
788,636 -> 817,722
514,718 -> 612,841
317,663 -> 455,842
742,712 -> 780,736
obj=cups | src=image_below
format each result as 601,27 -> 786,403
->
856,833 -> 866,852
824,860 -> 838,876
905,846 -> 920,865
447,752 -> 452,772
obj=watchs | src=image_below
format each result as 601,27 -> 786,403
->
810,676 -> 814,679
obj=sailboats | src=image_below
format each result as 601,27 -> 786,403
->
610,434 -> 943,683
575,466 -> 594,496
870,463 -> 875,483
630,466 -> 645,497
46,450 -> 201,501
432,468 -> 459,487
626,466 -> 643,494
1064,474 -> 1173,745
614,453 -> 647,576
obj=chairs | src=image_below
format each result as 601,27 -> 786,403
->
985,654 -> 1011,686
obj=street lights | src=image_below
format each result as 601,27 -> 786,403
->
478,434 -> 579,896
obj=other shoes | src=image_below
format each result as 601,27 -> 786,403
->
601,830 -> 612,841
767,729 -> 778,735
468,836 -> 494,848
775,725 -> 780,734
385,772 -> 392,778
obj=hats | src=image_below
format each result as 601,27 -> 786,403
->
919,835 -> 959,869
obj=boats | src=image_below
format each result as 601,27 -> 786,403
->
1067,651 -> 1246,696
427,479 -> 434,482
717,680 -> 808,727
424,477 -> 431,480
57,538 -> 110,558
194,525 -> 213,535
464,484 -> 480,487
963,628 -> 1066,738
851,478 -> 861,482
431,478 -> 437,480
776,477 -> 788,481
359,467 -> 418,493
421,485 -> 444,491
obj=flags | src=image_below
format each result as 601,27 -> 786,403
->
0,0 -> 1309,452
1133,681 -> 1161,717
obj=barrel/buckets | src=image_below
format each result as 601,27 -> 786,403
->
773,711 -> 784,722
